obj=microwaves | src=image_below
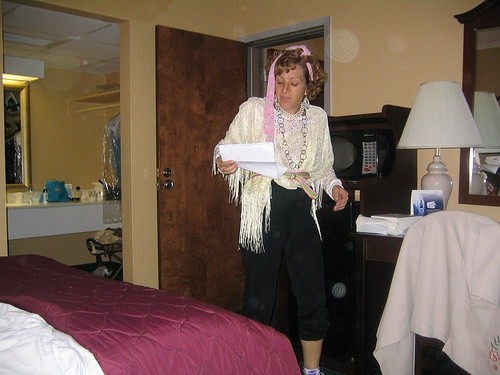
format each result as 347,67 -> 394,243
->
330,130 -> 394,180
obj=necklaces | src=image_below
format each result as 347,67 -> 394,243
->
274,101 -> 308,169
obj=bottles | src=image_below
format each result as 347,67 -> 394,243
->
75,186 -> 81,201
42,189 -> 48,203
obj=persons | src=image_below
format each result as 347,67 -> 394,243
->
213,45 -> 349,375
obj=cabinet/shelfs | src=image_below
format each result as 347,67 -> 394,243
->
287,105 -> 417,375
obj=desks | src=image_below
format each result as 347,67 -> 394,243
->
344,231 -> 403,375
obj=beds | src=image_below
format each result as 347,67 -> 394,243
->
0,254 -> 303,375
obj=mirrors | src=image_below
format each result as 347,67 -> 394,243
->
453,0 -> 500,206
3,79 -> 32,193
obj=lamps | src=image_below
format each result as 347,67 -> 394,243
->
3,56 -> 44,82
473,90 -> 500,195
396,81 -> 485,211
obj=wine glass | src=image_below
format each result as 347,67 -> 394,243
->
26,187 -> 34,203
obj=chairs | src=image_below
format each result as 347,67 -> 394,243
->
373,210 -> 500,375
